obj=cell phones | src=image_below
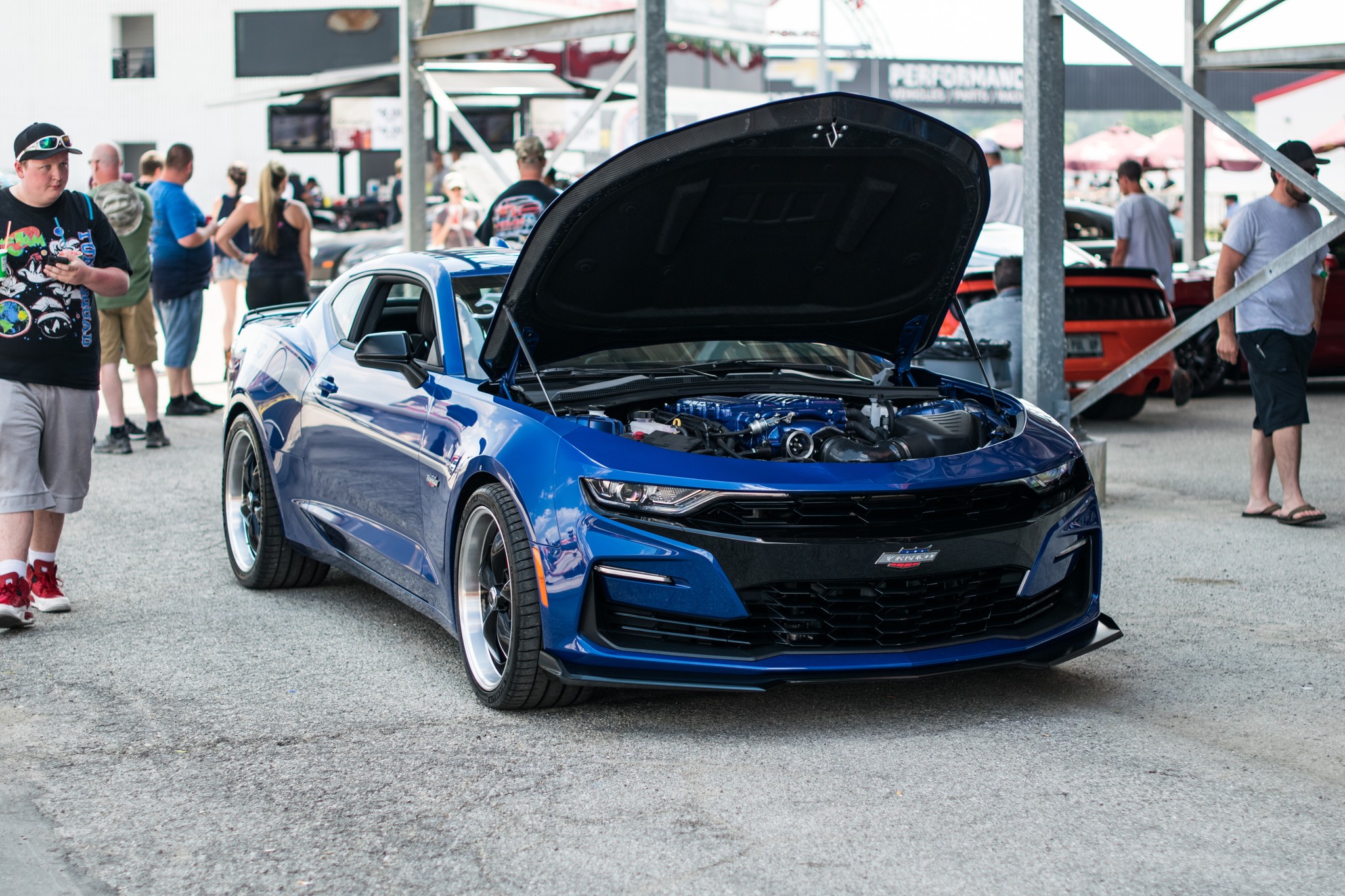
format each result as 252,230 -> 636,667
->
46,255 -> 69,267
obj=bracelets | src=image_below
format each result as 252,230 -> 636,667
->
234,253 -> 244,263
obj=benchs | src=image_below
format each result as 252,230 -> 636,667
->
374,304 -> 420,335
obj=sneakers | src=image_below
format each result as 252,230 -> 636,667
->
0,572 -> 36,626
27,559 -> 73,613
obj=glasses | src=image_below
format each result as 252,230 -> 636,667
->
1303,167 -> 1320,175
15,134 -> 73,161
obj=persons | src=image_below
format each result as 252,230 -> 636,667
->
85,143 -> 222,454
1222,192 -> 1241,225
431,171 -> 482,248
977,137 -> 1023,227
209,163 -> 251,381
214,161 -> 314,310
954,256 -> 1023,397
389,158 -> 404,228
288,172 -> 319,220
474,134 -> 561,246
1110,157 -> 1189,409
0,122 -> 132,630
427,139 -> 466,196
1214,141 -> 1332,525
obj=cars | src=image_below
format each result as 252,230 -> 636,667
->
935,218 -> 1176,423
1169,228 -> 1345,399
1061,195 -> 1210,268
217,88 -> 1122,715
308,196 -> 486,287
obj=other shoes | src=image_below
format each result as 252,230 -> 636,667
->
1173,368 -> 1192,407
93,435 -> 134,454
188,393 -> 225,412
166,401 -> 212,415
144,421 -> 169,449
125,418 -> 147,440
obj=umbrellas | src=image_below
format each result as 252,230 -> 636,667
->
1150,118 -> 1266,173
1063,124 -> 1186,176
981,118 -> 1026,151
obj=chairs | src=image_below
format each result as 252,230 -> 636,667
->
408,280 -> 491,362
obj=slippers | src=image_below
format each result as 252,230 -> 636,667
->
1277,504 -> 1327,524
1241,503 -> 1280,518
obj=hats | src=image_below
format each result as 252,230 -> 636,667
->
94,180 -> 145,237
13,122 -> 83,160
1270,141 -> 1330,171
976,136 -> 1001,154
514,135 -> 546,163
443,171 -> 464,190
